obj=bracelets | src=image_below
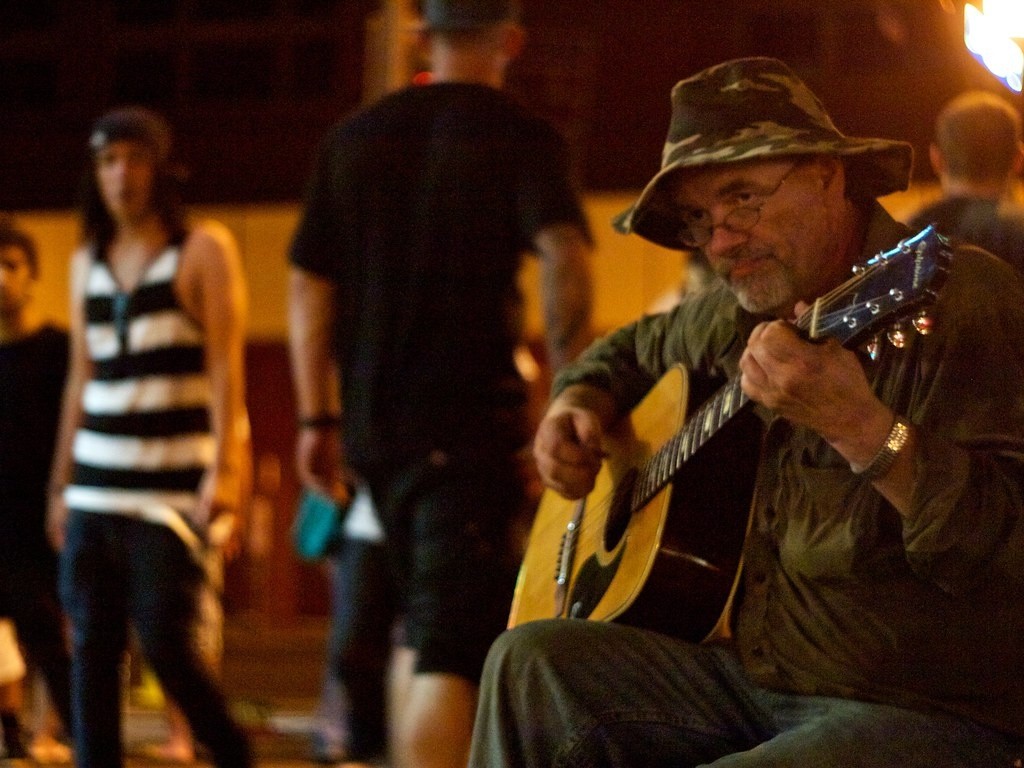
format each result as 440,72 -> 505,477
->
847,413 -> 913,483
295,412 -> 334,433
48,483 -> 64,496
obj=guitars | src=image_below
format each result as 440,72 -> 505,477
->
504,222 -> 957,644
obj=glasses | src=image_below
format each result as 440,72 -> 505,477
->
669,155 -> 812,249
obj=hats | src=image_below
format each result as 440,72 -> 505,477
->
610,57 -> 914,252
932,90 -> 1022,155
84,110 -> 171,169
419,0 -> 521,31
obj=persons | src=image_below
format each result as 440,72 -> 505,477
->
0,0 -> 598,768
470,54 -> 1024,768
907,92 -> 1024,271
